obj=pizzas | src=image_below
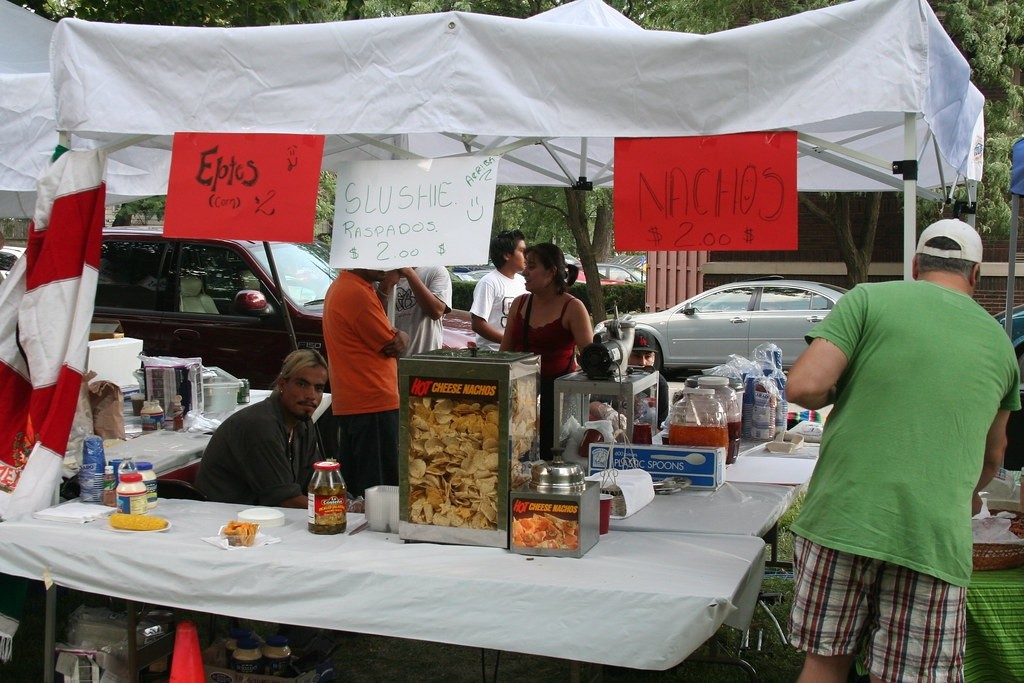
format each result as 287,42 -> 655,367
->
511,511 -> 578,551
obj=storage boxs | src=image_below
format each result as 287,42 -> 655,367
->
509,474 -> 601,560
89,317 -> 125,339
205,383 -> 237,412
392,352 -> 543,551
202,637 -> 319,683
54,642 -> 128,683
590,442 -> 727,490
86,337 -> 143,390
70,608 -> 168,671
555,364 -> 660,452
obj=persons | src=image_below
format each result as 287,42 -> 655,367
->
374,267 -> 452,369
322,267 -> 409,491
194,348 -> 365,651
588,327 -> 669,434
788,218 -> 1022,683
467,227 -> 532,353
499,241 -> 595,463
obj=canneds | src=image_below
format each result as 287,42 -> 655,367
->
116,461 -> 157,515
307,461 -> 347,535
140,400 -> 164,434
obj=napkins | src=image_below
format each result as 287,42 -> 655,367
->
30,500 -> 117,526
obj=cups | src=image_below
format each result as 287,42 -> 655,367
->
633,423 -> 653,445
578,429 -> 604,458
598,495 -> 614,534
741,349 -> 787,441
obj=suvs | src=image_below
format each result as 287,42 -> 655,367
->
89,226 -> 378,391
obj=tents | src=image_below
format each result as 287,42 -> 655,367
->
1005,137 -> 1023,340
0,2 -> 988,683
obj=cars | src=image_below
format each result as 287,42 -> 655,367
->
447,253 -> 647,285
594,280 -> 852,383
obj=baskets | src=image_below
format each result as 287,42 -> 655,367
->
598,428 -> 634,516
972,508 -> 1024,571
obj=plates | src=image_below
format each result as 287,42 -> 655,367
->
102,519 -> 172,533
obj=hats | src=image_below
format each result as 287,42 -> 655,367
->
631,329 -> 658,353
916,218 -> 983,264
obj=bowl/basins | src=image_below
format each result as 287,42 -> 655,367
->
364,485 -> 399,534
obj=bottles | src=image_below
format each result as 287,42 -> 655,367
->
260,635 -> 292,677
230,638 -> 262,673
131,394 -> 184,432
238,379 -> 251,403
669,374 -> 745,464
80,436 -> 157,516
308,461 -> 348,534
224,627 -> 252,668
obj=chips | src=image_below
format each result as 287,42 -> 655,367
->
408,374 -> 536,531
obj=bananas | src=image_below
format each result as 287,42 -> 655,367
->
110,513 -> 168,530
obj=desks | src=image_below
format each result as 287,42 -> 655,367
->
609,475 -> 801,566
0,495 -> 768,683
103,388 -> 334,488
651,427 -> 822,485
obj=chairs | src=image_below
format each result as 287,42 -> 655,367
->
175,277 -> 220,316
237,271 -> 274,316
